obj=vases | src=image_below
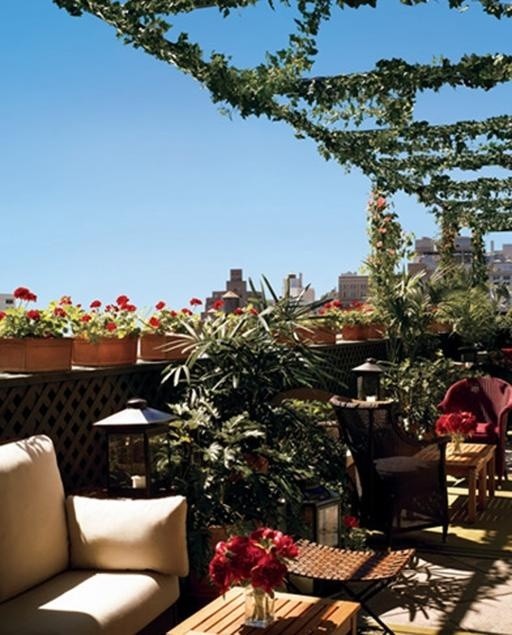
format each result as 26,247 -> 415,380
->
70,336 -> 98,367
345,322 -> 385,338
1,337 -> 26,370
21,336 -> 72,373
141,333 -> 194,361
451,435 -> 463,454
307,323 -> 336,344
98,337 -> 138,368
241,589 -> 277,630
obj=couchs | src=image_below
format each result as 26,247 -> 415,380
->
0,434 -> 188,635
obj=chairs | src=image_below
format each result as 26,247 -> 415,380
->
441,375 -> 512,480
331,399 -> 448,547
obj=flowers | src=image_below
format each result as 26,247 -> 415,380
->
362,192 -> 435,445
320,301 -> 379,321
434,414 -> 476,439
210,529 -> 296,613
143,296 -> 261,336
0,286 -> 137,337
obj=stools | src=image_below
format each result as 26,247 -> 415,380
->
280,538 -> 415,634
